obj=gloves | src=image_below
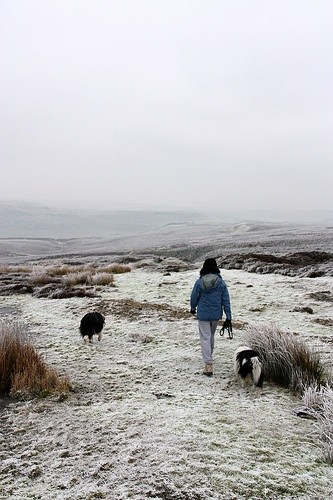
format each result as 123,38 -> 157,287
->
190,309 -> 196,314
223,319 -> 231,329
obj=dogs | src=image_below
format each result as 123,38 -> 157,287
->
235,347 -> 261,386
79,312 -> 105,342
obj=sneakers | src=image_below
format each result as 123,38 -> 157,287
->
203,363 -> 213,376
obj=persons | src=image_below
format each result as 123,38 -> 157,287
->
190,258 -> 231,376
